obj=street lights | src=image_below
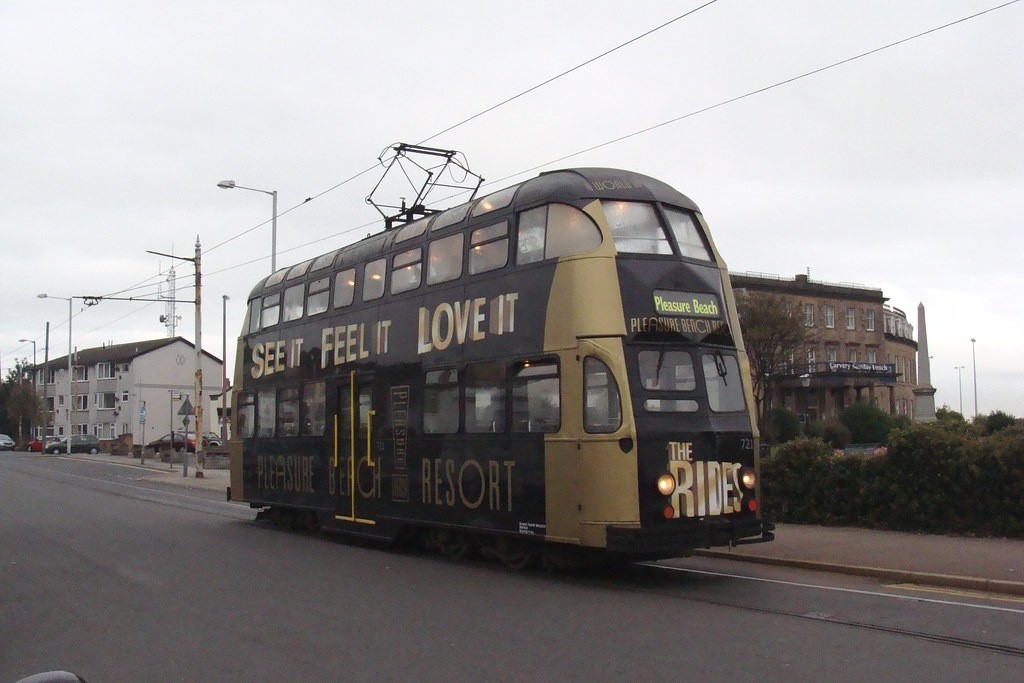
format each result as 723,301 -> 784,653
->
18,339 -> 36,396
955,366 -> 966,414
971,337 -> 978,418
37,292 -> 73,456
217,180 -> 278,274
221,293 -> 231,447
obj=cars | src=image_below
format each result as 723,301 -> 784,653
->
0,434 -> 16,451
145,430 -> 223,456
26,434 -> 65,452
46,433 -> 102,456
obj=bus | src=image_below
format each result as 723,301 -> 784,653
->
225,142 -> 776,580
